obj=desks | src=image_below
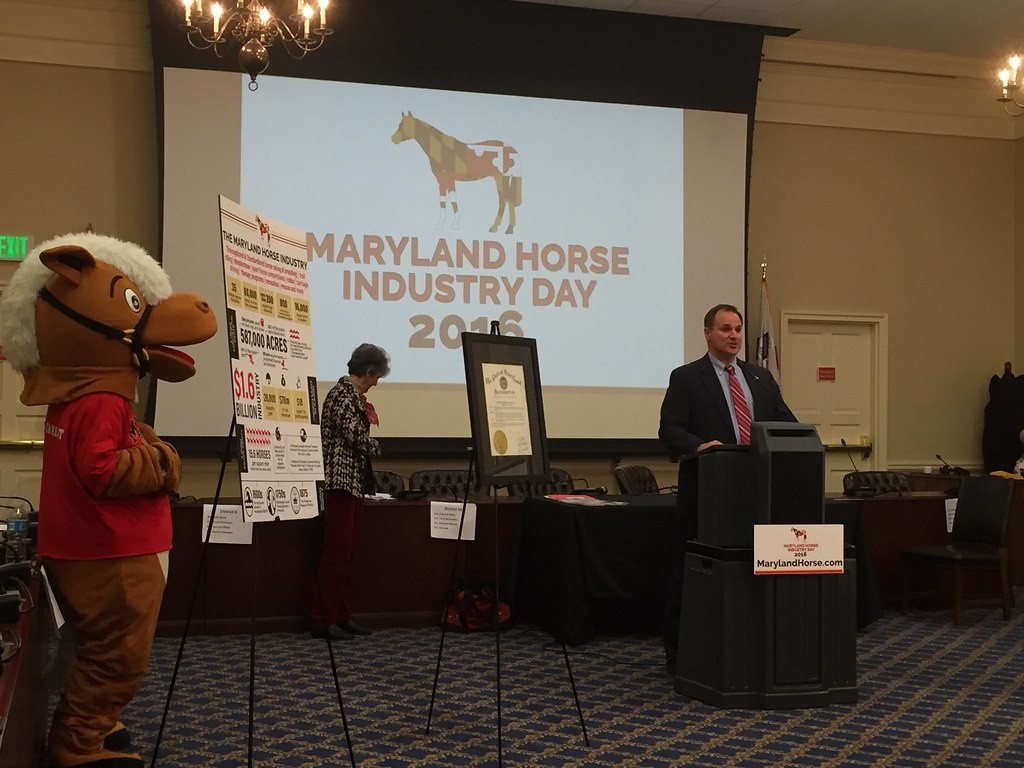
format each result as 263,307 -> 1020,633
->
832,492 -> 948,613
159,487 -> 686,654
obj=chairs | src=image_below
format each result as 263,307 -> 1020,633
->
514,468 -> 609,497
617,465 -> 680,498
407,470 -> 482,496
904,473 -> 1012,630
371,471 -> 407,498
843,470 -> 912,494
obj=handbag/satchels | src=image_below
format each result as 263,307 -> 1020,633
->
442,583 -> 512,632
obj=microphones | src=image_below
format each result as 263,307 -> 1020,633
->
736,361 -> 759,381
936,455 -> 958,474
840,439 -> 877,498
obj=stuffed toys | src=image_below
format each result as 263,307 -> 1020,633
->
0,231 -> 218,768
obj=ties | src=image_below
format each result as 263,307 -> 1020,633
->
723,363 -> 752,445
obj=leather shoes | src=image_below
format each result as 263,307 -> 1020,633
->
312,621 -> 372,640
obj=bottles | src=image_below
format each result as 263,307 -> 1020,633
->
6,502 -> 29,563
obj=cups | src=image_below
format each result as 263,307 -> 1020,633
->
923,466 -> 932,473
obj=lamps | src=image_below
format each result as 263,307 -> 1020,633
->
182,0 -> 333,88
997,55 -> 1024,116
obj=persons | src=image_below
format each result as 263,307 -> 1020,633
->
309,344 -> 389,639
658,304 -> 798,673
